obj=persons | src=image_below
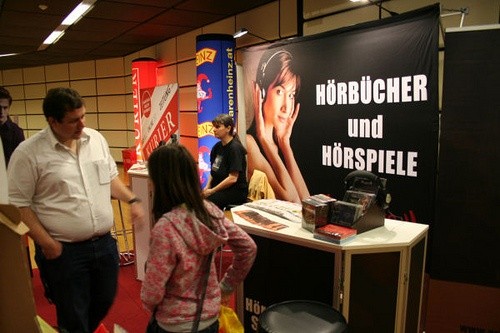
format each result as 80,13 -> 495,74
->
6,87 -> 146,333
201,113 -> 249,211
244,43 -> 310,204
140,143 -> 258,333
0,85 -> 26,170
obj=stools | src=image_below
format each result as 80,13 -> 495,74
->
110,186 -> 135,266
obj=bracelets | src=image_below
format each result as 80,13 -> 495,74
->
128,196 -> 142,206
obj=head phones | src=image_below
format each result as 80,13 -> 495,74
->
343,170 -> 387,208
254,50 -> 293,102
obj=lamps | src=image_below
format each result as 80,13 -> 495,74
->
233,27 -> 269,42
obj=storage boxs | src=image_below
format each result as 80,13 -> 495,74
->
331,187 -> 387,230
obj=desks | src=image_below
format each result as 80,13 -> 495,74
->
231,200 -> 428,333
127,164 -> 153,283
